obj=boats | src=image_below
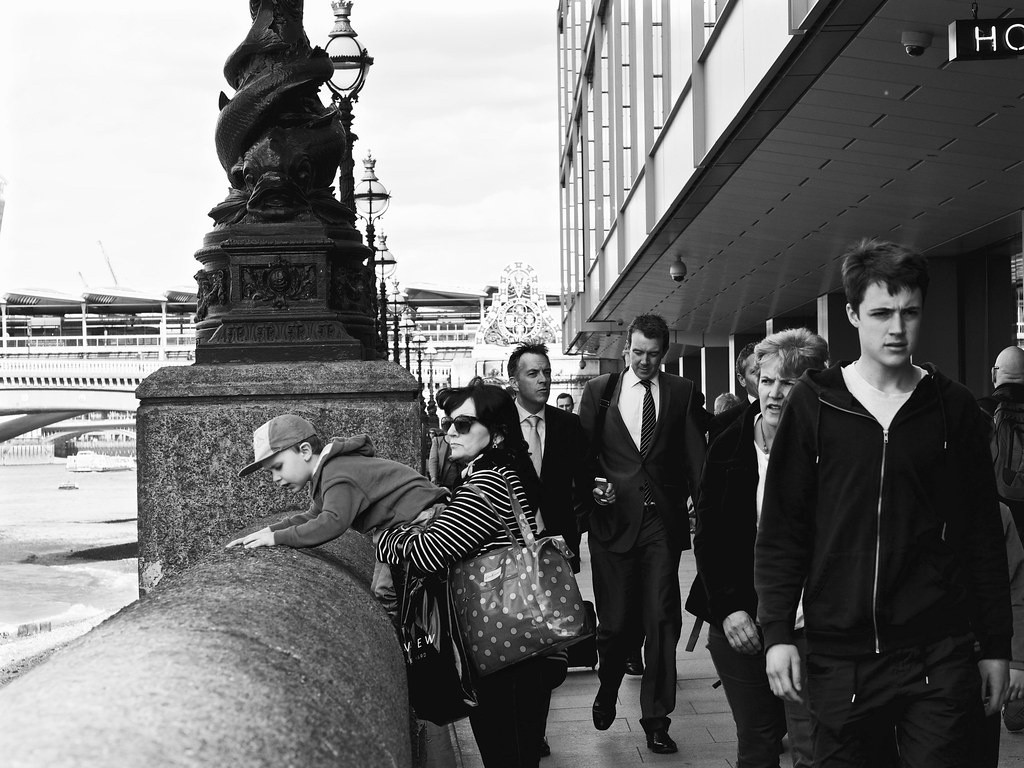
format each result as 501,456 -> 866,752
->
66,450 -> 136,472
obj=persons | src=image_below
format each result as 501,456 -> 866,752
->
507,344 -> 615,574
429,436 -> 452,485
582,317 -> 707,754
707,342 -> 758,443
755,243 -> 1011,768
226,415 -> 453,646
981,346 -> 1024,729
684,328 -> 829,768
558,393 -> 574,413
371,385 -> 568,767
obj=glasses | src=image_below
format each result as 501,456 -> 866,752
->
441,416 -> 485,436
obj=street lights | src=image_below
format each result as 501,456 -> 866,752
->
374,228 -> 397,361
386,277 -> 407,365
321,0 -> 375,215
355,148 -> 392,336
398,315 -> 416,372
411,333 -> 428,418
423,345 -> 440,429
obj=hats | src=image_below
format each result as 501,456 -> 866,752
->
238,414 -> 317,476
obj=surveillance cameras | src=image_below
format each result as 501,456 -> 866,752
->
900,32 -> 932,57
670,261 -> 687,282
579,361 -> 586,370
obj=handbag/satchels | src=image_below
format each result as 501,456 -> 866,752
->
445,470 -> 593,680
396,545 -> 479,726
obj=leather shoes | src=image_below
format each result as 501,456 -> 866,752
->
625,645 -> 644,676
592,681 -> 620,731
646,731 -> 678,755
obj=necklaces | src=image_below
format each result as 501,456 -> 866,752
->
761,420 -> 767,454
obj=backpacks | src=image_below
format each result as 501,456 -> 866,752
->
988,394 -> 1024,506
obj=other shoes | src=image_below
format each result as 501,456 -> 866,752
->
540,737 -> 549,757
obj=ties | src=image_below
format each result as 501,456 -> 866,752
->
640,379 -> 653,505
525,415 -> 544,534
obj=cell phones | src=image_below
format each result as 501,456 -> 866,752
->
595,477 -> 608,502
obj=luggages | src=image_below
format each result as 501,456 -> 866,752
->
566,601 -> 598,670
